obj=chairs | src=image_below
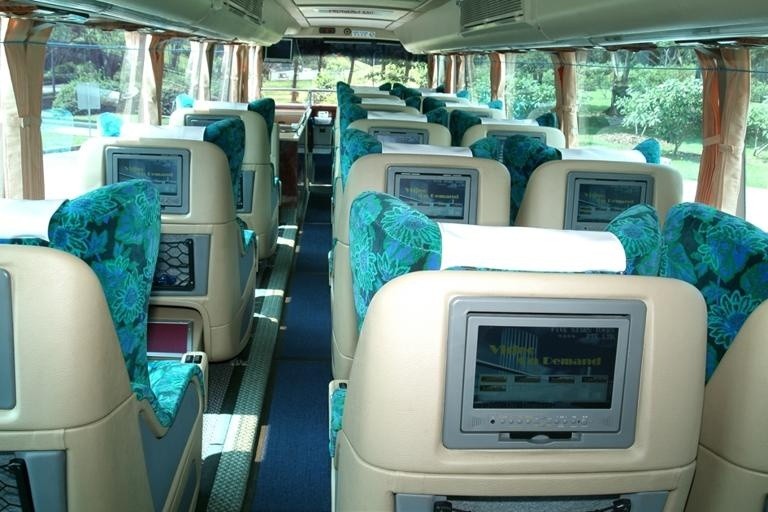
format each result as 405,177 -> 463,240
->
329,80 -> 767,510
1,93 -> 314,512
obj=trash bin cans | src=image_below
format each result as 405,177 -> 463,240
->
312,110 -> 333,146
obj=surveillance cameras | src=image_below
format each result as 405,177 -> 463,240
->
343,27 -> 351,35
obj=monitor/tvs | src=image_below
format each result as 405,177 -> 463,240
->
394,173 -> 470,224
460,315 -> 631,433
493,135 -> 540,164
263,39 -> 293,63
571,178 -> 647,231
374,132 -> 423,144
113,153 -> 182,206
